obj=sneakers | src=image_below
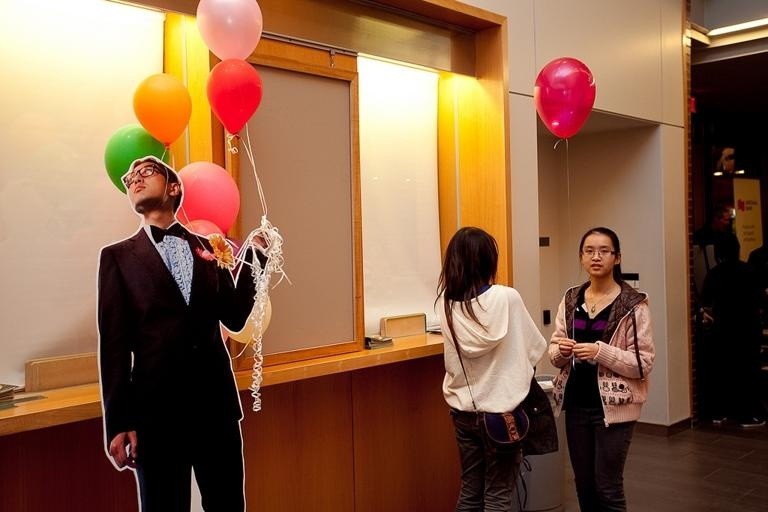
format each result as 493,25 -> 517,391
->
711,415 -> 729,425
738,417 -> 767,430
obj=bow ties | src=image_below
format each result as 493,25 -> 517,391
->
150,222 -> 189,245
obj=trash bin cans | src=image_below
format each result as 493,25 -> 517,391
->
513,374 -> 566,511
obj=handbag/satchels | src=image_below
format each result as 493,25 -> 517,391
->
514,375 -> 561,458
473,407 -> 531,461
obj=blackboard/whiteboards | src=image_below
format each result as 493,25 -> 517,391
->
0,0 -> 440,388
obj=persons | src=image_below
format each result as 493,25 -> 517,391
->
96,157 -> 274,511
549,227 -> 656,512
434,225 -> 547,511
691,205 -> 766,429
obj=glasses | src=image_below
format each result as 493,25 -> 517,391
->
581,246 -> 619,260
122,163 -> 167,188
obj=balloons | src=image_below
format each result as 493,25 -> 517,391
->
104,124 -> 170,195
196,0 -> 265,136
534,57 -> 596,138
174,159 -> 241,236
183,220 -> 271,343
133,73 -> 191,150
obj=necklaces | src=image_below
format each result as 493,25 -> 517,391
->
586,283 -> 614,313
